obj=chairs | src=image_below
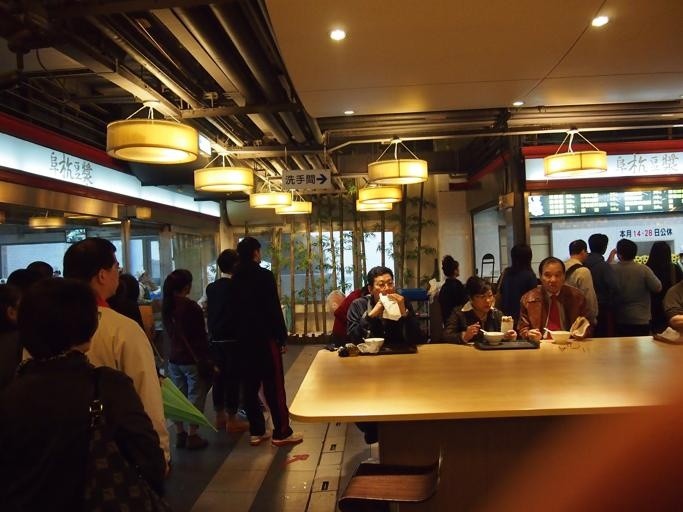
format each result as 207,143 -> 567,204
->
398,287 -> 432,343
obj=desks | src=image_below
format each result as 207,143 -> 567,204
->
287,331 -> 680,511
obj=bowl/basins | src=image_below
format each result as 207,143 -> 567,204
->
483,332 -> 503,345
364,338 -> 384,352
551,330 -> 571,344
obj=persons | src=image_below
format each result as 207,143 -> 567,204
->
138,271 -> 158,303
161,268 -> 219,450
204,249 -> 249,434
108,274 -> 145,332
0,261 -> 60,390
62,236 -> 171,479
226,237 -> 303,444
0,277 -> 165,511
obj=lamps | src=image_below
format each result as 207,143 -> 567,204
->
345,136 -> 429,216
95,215 -> 131,226
25,208 -> 67,231
98,95 -> 314,216
541,128 -> 608,178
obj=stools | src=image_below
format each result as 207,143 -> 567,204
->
333,453 -> 445,510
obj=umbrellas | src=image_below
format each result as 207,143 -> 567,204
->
159,376 -> 218,433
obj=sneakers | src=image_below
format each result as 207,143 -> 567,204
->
216,413 -> 229,429
225,414 -> 250,433
248,430 -> 272,443
185,434 -> 210,451
175,430 -> 189,449
272,432 -> 303,442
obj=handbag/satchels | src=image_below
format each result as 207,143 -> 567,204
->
83,366 -> 171,512
196,360 -> 213,376
